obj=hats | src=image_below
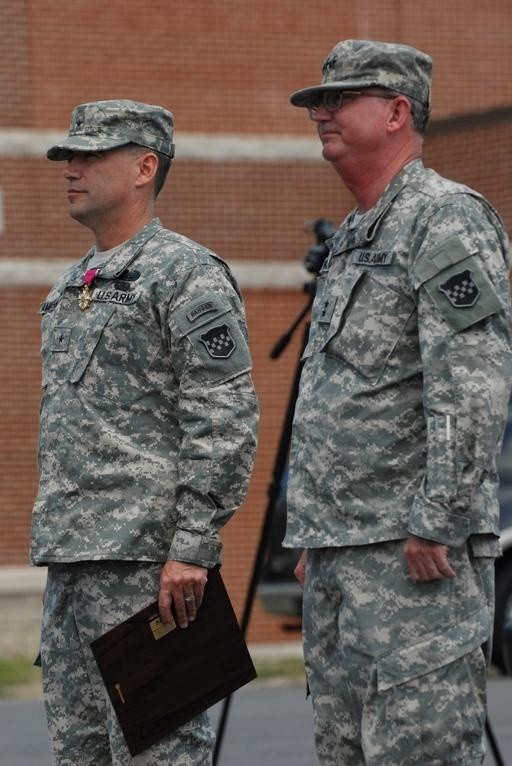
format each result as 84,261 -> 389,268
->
290,39 -> 433,108
46,99 -> 175,161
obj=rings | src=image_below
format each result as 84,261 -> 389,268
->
186,597 -> 193,603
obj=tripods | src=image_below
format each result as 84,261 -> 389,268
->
211,277 -> 506,766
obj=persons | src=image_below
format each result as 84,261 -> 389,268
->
29,103 -> 260,766
281,38 -> 511,765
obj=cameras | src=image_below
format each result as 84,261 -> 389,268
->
302,219 -> 339,273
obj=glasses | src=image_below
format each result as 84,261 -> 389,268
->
306,90 -> 414,115
